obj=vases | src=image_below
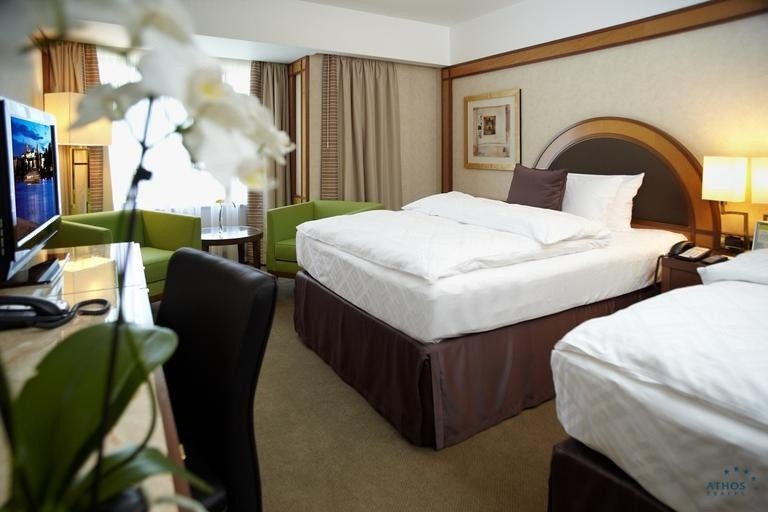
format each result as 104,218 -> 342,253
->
217,206 -> 222,233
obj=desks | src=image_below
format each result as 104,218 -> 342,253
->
200,226 -> 263,270
0,241 -> 192,512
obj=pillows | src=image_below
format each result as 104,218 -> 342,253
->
507,162 -> 567,211
562,170 -> 646,231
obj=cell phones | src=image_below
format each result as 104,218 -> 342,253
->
702,254 -> 729,265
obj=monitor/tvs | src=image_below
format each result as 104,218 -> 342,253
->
0,95 -> 70,288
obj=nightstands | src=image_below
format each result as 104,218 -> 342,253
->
661,248 -> 739,293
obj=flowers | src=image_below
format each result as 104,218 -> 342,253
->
16,0 -> 297,511
215,198 -> 224,206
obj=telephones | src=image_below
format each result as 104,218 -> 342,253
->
0,295 -> 69,331
668,242 -> 712,262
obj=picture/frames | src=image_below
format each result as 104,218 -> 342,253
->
752,221 -> 768,250
463,89 -> 521,171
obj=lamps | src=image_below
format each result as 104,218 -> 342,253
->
42,92 -> 112,215
702,156 -> 750,251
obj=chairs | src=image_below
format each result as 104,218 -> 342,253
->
267,200 -> 384,279
46,210 -> 202,304
155,247 -> 278,512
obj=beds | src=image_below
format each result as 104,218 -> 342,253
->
548,249 -> 768,512
295,117 -> 721,452
